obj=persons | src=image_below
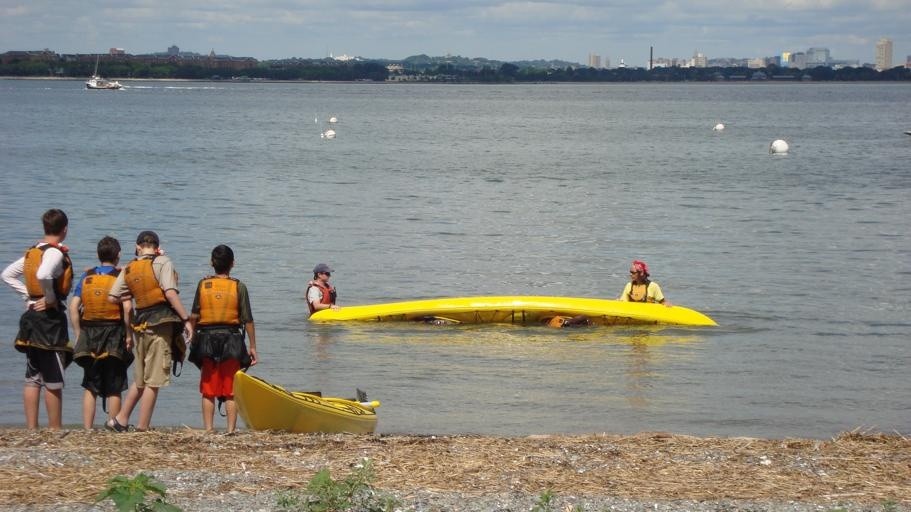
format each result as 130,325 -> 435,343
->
68,236 -> 136,433
185,243 -> 256,434
306,264 -> 339,315
0,209 -> 76,432
618,260 -> 671,307
104,231 -> 193,433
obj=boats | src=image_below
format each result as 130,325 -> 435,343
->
310,329 -> 708,361
307,294 -> 720,328
231,369 -> 379,434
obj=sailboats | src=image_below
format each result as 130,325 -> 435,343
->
85,53 -> 122,91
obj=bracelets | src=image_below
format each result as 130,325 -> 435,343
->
329,304 -> 331,309
182,316 -> 190,322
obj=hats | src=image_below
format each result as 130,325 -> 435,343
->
630,260 -> 650,277
134,230 -> 160,256
313,262 -> 335,275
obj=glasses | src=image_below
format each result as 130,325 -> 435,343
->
322,272 -> 330,277
630,270 -> 640,274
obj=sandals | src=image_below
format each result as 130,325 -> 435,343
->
104,415 -> 129,435
